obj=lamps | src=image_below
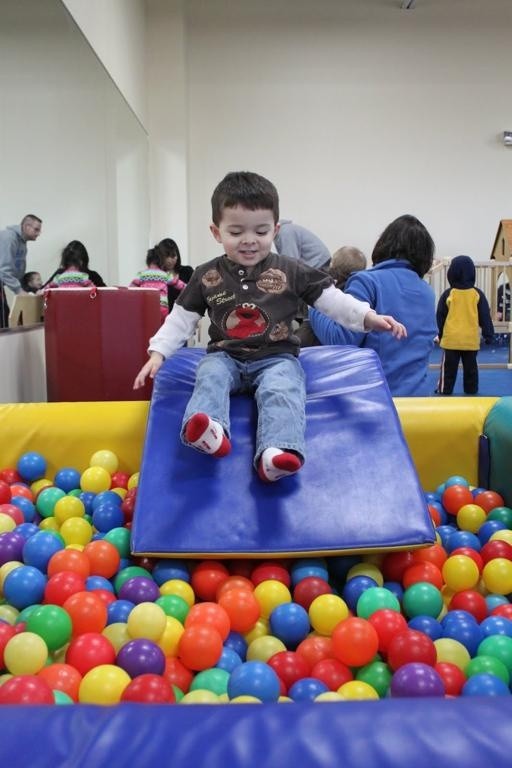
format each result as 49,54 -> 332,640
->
503,130 -> 512,146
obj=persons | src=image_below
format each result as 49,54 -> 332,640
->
133,172 -> 408,481
437,254 -> 494,395
128,249 -> 186,317
43,246 -> 93,288
331,247 -> 367,283
274,219 -> 331,275
1,214 -> 43,328
309,215 -> 436,396
46,240 -> 106,290
23,271 -> 44,290
157,239 -> 195,311
